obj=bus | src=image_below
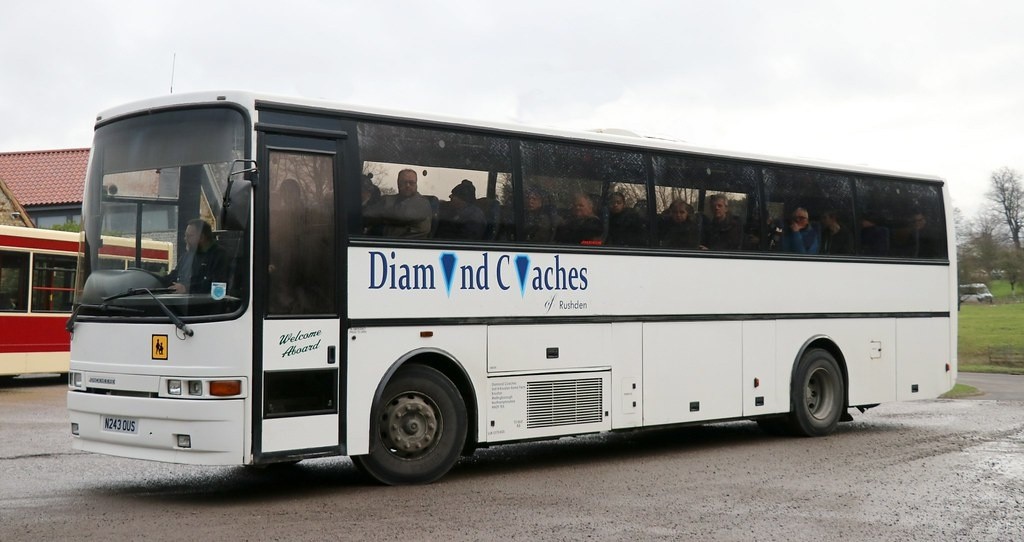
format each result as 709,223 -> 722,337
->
64,93 -> 961,485
0,225 -> 174,382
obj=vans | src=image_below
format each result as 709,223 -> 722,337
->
959,283 -> 993,304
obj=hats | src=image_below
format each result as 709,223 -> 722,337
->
451,179 -> 477,204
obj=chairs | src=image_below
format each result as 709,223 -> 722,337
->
349,187 -> 891,257
212,229 -> 245,294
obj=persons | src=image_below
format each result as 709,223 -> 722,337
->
659,199 -> 703,250
907,215 -> 936,257
268,179 -> 320,288
855,212 -> 890,257
742,186 -> 774,248
600,192 -> 652,249
777,207 -> 818,254
362,170 -> 433,237
153,220 -> 233,296
361,174 -> 381,190
510,189 -> 555,244
555,194 -> 605,245
819,209 -> 852,254
436,182 -> 487,238
702,194 -> 741,251
